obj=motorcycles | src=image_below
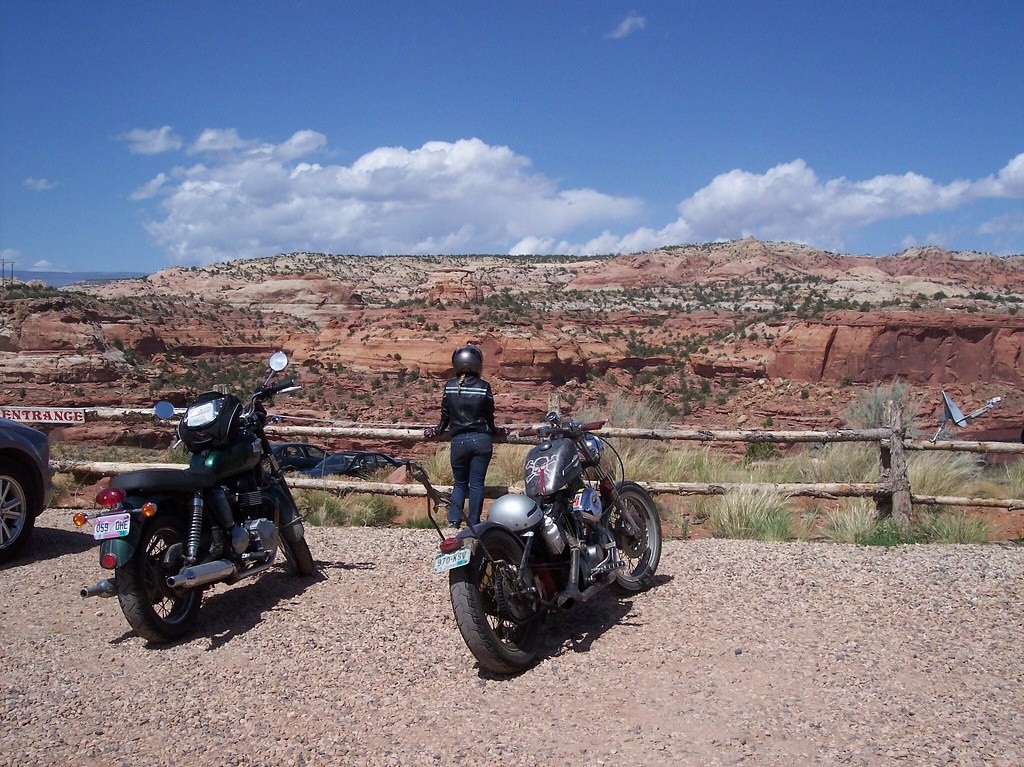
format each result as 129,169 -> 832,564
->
404,412 -> 662,674
72,351 -> 317,644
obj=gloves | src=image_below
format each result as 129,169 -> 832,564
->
423,427 -> 437,438
492,426 -> 510,437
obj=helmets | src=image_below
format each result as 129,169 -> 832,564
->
486,494 -> 544,532
452,344 -> 482,378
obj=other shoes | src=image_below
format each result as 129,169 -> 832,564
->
449,521 -> 460,529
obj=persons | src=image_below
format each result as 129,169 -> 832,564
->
424,344 -> 511,530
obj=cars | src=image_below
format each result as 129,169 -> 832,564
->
0,417 -> 54,565
254,442 -> 330,472
301,449 -> 406,480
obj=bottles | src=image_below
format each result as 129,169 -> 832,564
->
542,516 -> 565,555
